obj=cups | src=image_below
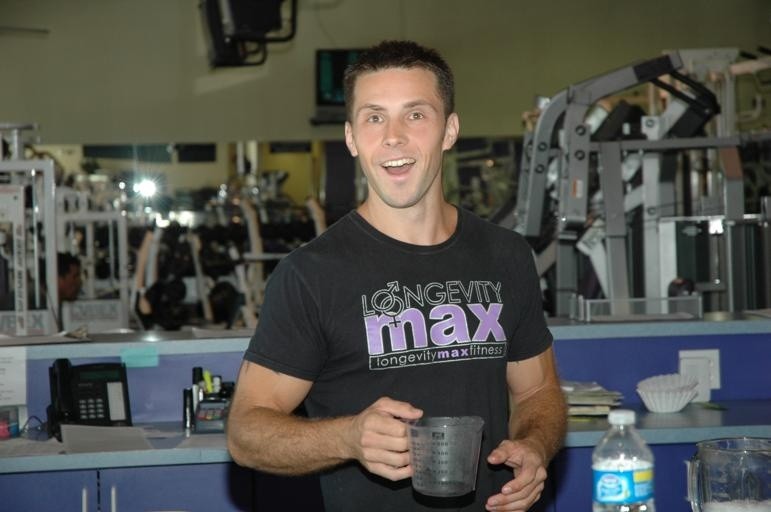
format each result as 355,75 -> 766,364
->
398,417 -> 486,498
686,437 -> 770,512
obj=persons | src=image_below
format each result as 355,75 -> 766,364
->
224,38 -> 569,511
17,252 -> 87,329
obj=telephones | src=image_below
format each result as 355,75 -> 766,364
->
48,358 -> 132,443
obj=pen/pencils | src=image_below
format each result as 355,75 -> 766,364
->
202,369 -> 222,394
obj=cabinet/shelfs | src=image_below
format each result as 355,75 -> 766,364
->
0,461 -> 237,512
546,446 -> 768,512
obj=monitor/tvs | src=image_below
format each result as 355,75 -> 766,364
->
197,0 -> 242,66
314,47 -> 372,122
218,0 -> 281,40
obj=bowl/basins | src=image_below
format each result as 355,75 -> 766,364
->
636,372 -> 700,413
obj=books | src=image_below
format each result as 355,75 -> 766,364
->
556,378 -> 624,417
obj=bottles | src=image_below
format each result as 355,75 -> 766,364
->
590,409 -> 655,512
